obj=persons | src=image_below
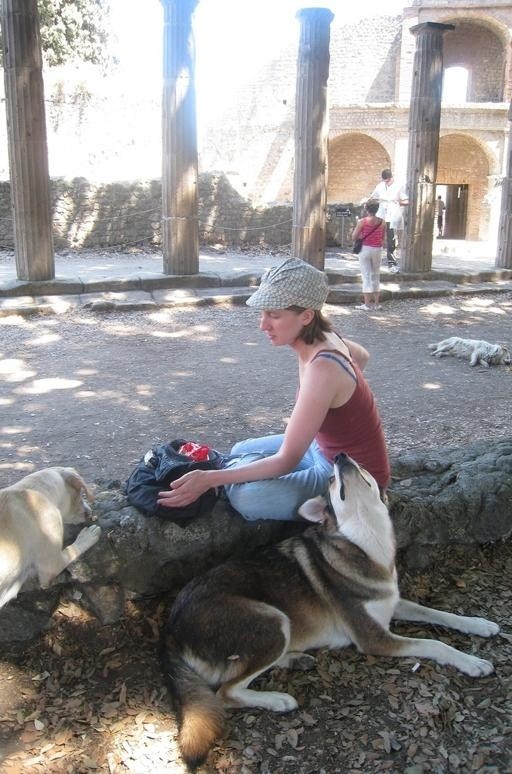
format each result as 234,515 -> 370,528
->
154,256 -> 393,524
349,198 -> 388,315
360,169 -> 409,274
436,195 -> 446,238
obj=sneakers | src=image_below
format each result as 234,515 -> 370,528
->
354,304 -> 382,311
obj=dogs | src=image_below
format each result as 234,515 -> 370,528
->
156,450 -> 501,767
0,466 -> 102,609
426,337 -> 512,369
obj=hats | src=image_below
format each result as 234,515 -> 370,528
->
245,257 -> 330,312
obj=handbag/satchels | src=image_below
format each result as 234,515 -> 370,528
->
124,439 -> 226,530
352,238 -> 362,254
